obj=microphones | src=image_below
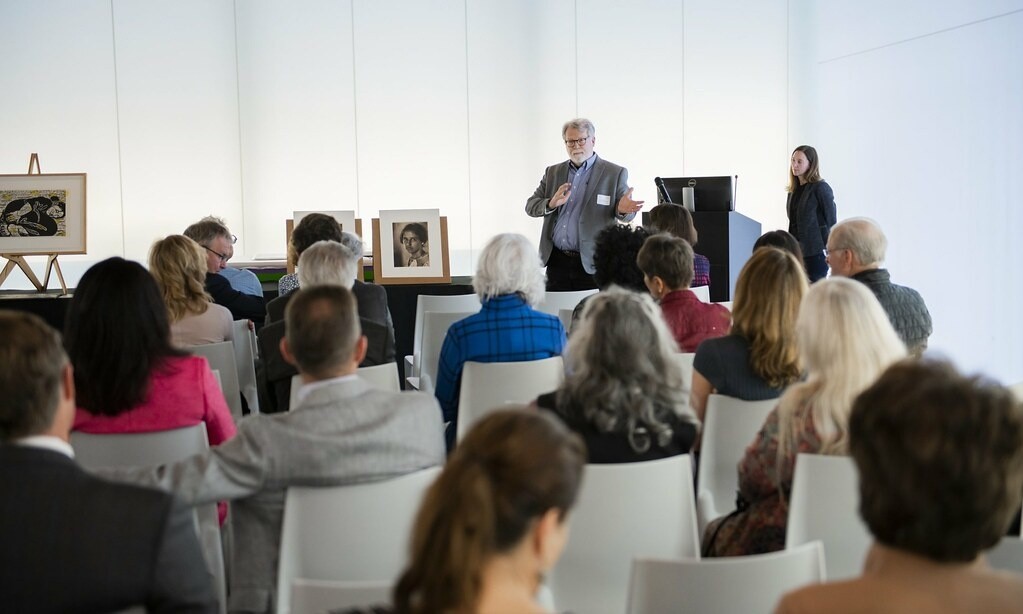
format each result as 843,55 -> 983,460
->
655,177 -> 672,204
733,175 -> 738,211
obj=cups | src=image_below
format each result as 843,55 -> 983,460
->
683,187 -> 694,211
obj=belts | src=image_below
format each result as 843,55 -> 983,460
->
562,250 -> 580,257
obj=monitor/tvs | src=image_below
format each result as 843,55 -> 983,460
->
658,175 -> 732,211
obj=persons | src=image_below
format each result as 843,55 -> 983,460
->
525,117 -> 645,293
0,203 -> 1023,614
784,145 -> 837,283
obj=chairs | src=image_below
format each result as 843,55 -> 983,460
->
58,286 -> 1023,614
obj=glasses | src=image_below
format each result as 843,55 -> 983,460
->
822,247 -> 848,257
229,235 -> 237,244
564,135 -> 590,147
200,244 -> 226,262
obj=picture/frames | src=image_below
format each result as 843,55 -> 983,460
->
378,209 -> 443,278
292,210 -> 355,274
0,173 -> 87,256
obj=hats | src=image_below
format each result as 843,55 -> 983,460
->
293,213 -> 340,257
